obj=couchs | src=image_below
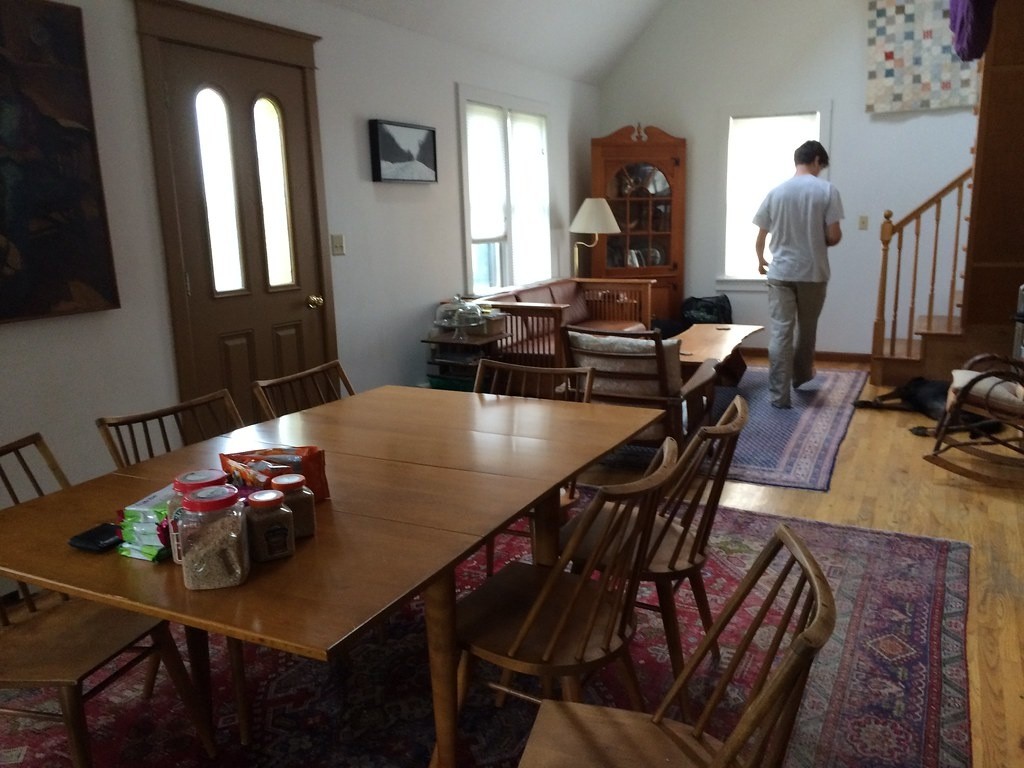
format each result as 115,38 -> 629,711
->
440,275 -> 658,394
555,320 -> 717,461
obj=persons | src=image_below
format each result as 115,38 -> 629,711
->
753,139 -> 845,409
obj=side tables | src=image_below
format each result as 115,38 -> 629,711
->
421,329 -> 515,391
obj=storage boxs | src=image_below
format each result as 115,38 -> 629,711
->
468,315 -> 507,334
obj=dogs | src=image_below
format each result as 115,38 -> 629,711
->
854,377 -> 1004,439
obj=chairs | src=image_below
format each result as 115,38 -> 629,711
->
250,360 -> 356,419
428,436 -> 678,768
923,352 -> 1024,490
0,429 -> 223,768
95,387 -> 253,748
474,357 -> 599,575
519,522 -> 841,768
557,393 -> 749,724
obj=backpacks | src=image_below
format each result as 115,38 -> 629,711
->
680,294 -> 747,389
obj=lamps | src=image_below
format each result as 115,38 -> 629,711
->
569,197 -> 621,274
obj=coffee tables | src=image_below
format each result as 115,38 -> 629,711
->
667,323 -> 767,429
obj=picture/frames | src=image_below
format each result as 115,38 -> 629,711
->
367,119 -> 438,183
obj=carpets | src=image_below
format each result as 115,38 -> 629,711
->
596,365 -> 871,492
0,485 -> 974,768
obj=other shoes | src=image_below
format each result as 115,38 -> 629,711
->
793,368 -> 816,389
771,402 -> 793,410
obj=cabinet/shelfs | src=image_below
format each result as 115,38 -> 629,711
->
590,118 -> 686,343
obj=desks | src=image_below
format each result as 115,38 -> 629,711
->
0,383 -> 668,768
